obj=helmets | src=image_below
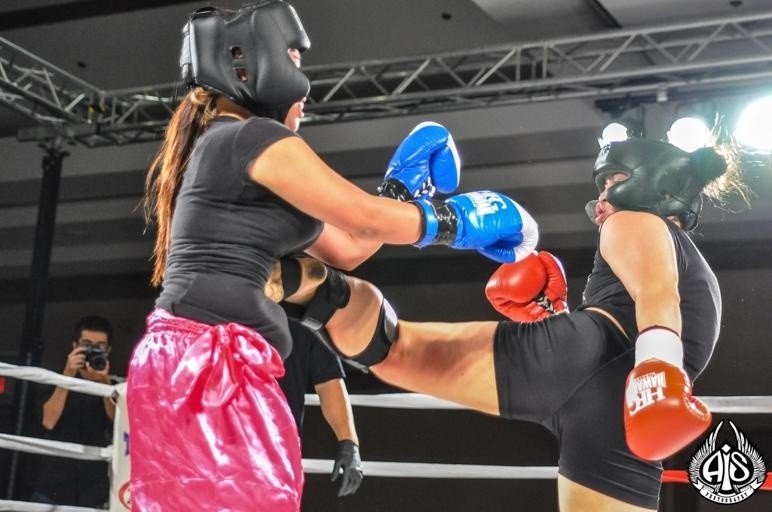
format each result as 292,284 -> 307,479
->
179,0 -> 311,123
594,103 -> 727,230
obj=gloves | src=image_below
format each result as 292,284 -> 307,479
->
377,121 -> 569,322
331,439 -> 364,496
624,358 -> 711,462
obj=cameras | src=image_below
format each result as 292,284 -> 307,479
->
83,349 -> 107,371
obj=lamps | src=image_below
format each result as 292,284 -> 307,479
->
593,95 -> 716,152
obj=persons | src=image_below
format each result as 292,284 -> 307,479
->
272,308 -> 364,499
121,1 -> 541,512
262,136 -> 726,511
29,315 -> 118,511
